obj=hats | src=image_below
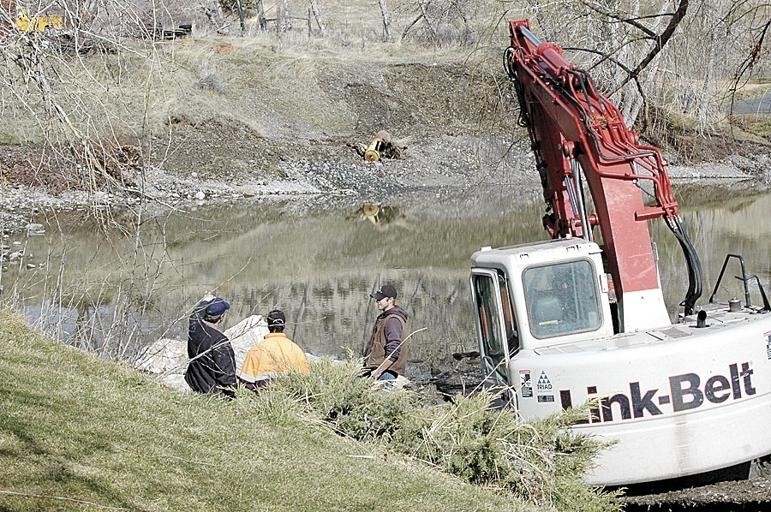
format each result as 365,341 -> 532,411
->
370,286 -> 397,300
206,298 -> 230,315
267,310 -> 286,321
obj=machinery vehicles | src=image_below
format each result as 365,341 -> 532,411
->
12,3 -> 62,37
455,12 -> 770,493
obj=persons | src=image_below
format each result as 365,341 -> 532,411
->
182,290 -> 236,400
236,309 -> 310,391
359,285 -> 408,381
